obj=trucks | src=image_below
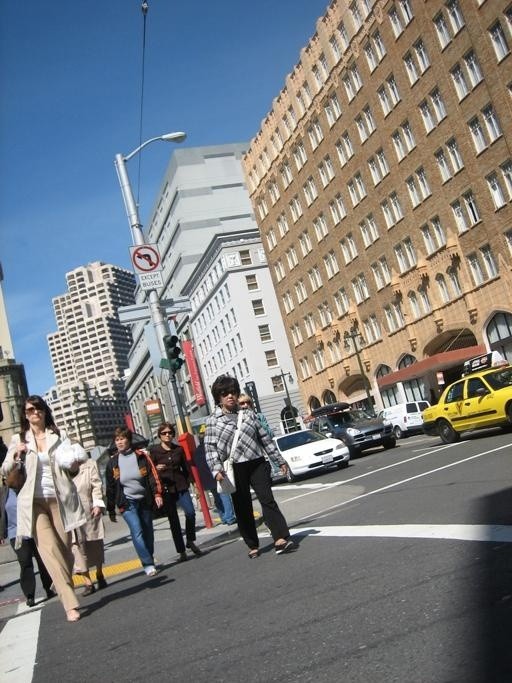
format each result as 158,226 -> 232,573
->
376,400 -> 431,439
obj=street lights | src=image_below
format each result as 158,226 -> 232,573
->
274,369 -> 300,431
114,126 -> 190,446
342,328 -> 377,415
72,379 -> 105,449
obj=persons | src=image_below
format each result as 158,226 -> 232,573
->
1,393 -> 105,622
149,422 -> 201,561
192,375 -> 294,559
105,425 -> 164,576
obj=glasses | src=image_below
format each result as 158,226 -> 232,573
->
24,406 -> 43,415
221,389 -> 236,397
160,431 -> 173,435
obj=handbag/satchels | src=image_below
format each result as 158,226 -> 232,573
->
217,461 -> 237,494
5,463 -> 26,488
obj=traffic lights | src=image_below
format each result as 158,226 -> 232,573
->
164,334 -> 183,374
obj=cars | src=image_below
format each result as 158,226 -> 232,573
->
268,429 -> 354,481
417,350 -> 512,445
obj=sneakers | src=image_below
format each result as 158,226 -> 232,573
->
66,609 -> 81,621
83,584 -> 94,596
187,542 -> 202,555
275,541 -> 294,554
249,550 -> 258,558
177,552 -> 187,561
27,598 -> 34,606
145,565 -> 157,577
97,574 -> 107,589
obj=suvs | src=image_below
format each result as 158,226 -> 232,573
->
304,402 -> 397,460
